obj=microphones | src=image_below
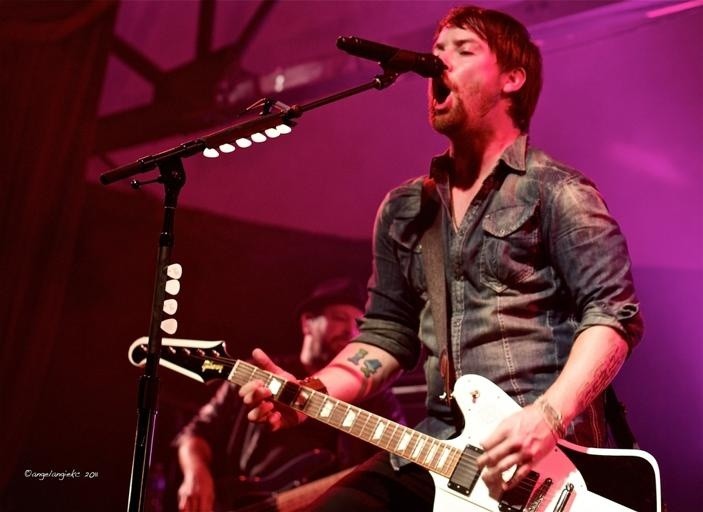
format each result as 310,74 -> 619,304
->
336,35 -> 443,78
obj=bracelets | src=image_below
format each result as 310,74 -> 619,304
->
534,395 -> 568,439
298,376 -> 330,396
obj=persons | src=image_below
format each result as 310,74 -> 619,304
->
238,5 -> 645,512
172,276 -> 410,512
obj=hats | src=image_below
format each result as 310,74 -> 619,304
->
290,277 -> 364,322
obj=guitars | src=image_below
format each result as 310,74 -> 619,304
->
128,336 -> 638,512
